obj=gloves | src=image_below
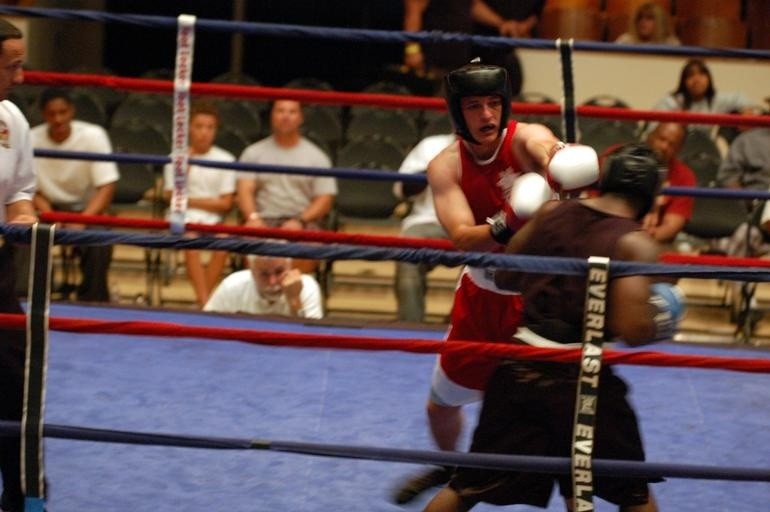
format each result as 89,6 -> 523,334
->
650,282 -> 683,321
545,141 -> 599,193
487,172 -> 550,244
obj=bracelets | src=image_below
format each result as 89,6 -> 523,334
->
406,43 -> 421,55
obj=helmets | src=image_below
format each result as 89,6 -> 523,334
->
600,144 -> 658,221
443,65 -> 510,145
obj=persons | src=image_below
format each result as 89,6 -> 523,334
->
394,134 -> 459,321
474,0 -> 547,42
598,120 -> 695,285
239,98 -> 338,274
164,104 -> 237,308
402,0 -> 472,96
718,125 -> 770,209
202,239 -> 323,321
613,0 -> 681,49
394,61 -> 600,504
30,87 -> 121,303
421,143 -> 686,512
657,59 -> 770,188
0,19 -> 39,314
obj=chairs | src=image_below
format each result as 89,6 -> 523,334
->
73,93 -> 103,125
354,85 -> 419,120
427,92 -> 447,118
272,107 -> 339,140
576,97 -> 637,132
108,126 -> 154,203
193,96 -> 262,144
245,132 -> 339,273
538,115 -> 565,140
423,116 -> 461,139
73,59 -> 119,105
213,127 -> 245,156
512,91 -> 562,124
129,91 -> 173,107
673,130 -> 746,257
212,73 -> 270,110
284,81 -> 340,112
335,140 -> 403,216
112,101 -> 170,144
579,122 -> 635,156
350,109 -> 412,144
144,68 -> 176,84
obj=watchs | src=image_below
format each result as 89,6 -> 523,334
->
298,309 -> 304,316
246,213 -> 260,222
